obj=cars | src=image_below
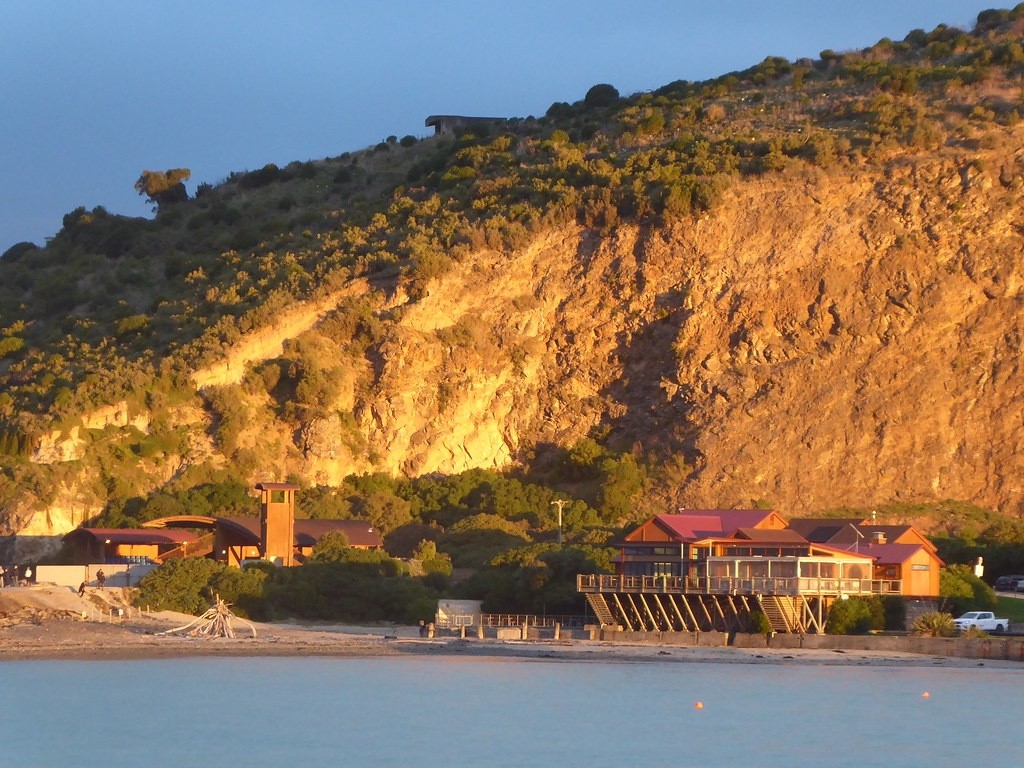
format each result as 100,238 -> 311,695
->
995,575 -> 1024,591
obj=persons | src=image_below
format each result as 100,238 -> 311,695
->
11,564 -> 19,586
0,564 -> 10,587
96,569 -> 106,591
428,619 -> 434,637
418,619 -> 425,637
78,581 -> 86,598
24,566 -> 32,587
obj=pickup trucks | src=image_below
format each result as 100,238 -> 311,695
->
952,611 -> 1010,637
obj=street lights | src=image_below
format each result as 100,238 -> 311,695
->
550,497 -> 568,545
